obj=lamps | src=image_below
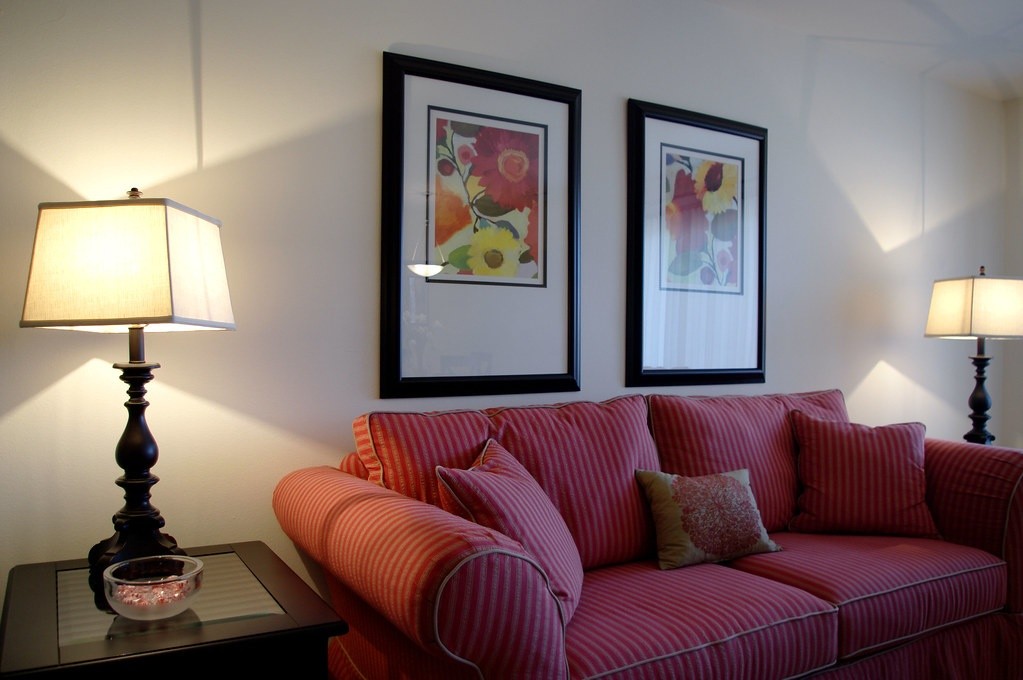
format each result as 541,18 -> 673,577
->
924,266 -> 1023,444
20,187 -> 237,616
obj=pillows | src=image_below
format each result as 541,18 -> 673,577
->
636,467 -> 785,570
788,408 -> 942,539
436,439 -> 584,626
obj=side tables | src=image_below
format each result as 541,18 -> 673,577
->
0,540 -> 349,680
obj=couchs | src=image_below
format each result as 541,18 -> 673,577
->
271,394 -> 1023,680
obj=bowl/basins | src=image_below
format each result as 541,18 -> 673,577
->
102,555 -> 205,621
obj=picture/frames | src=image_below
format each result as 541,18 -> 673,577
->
379,51 -> 582,398
625,98 -> 769,386
